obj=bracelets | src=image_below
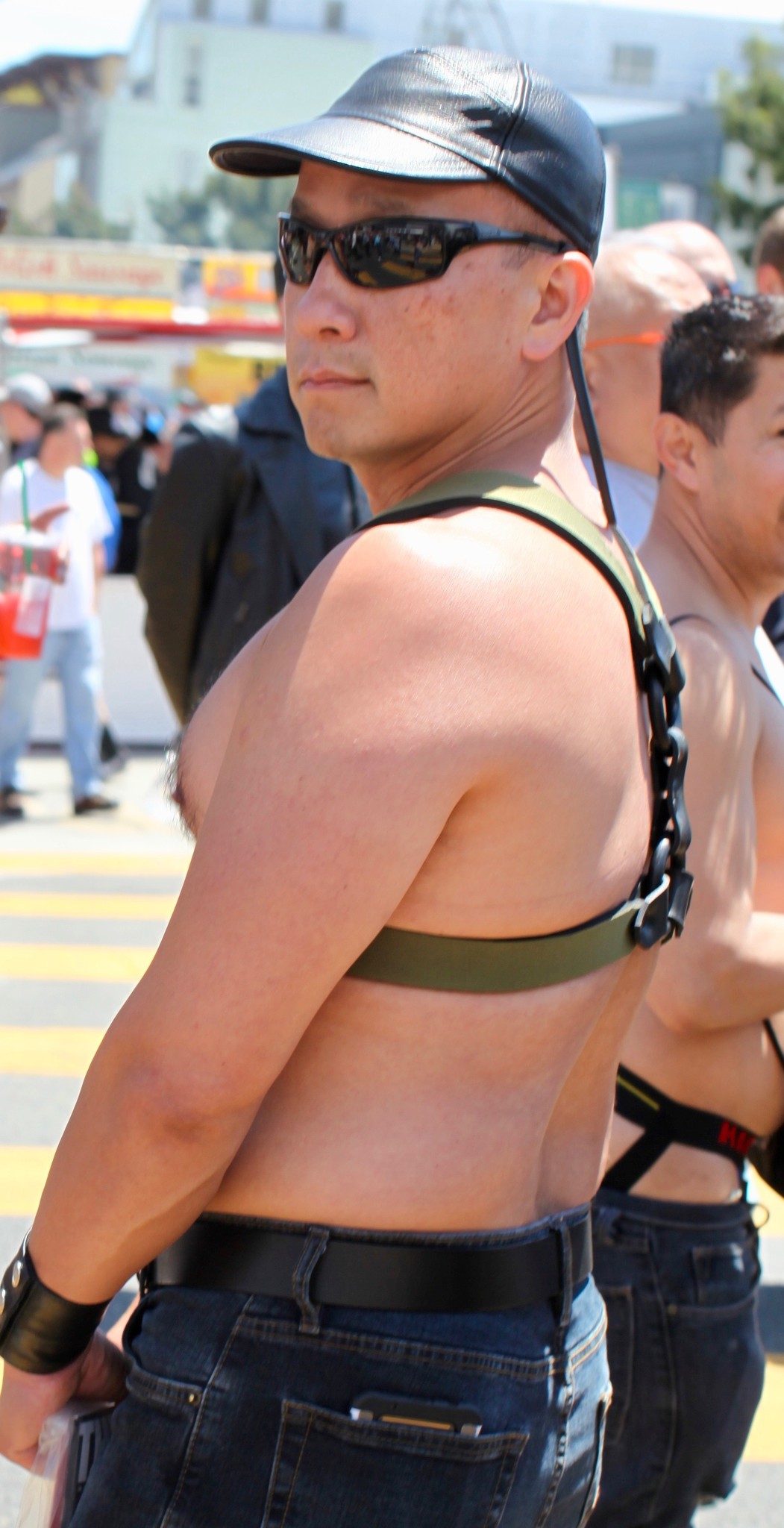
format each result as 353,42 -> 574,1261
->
0,1228 -> 116,1376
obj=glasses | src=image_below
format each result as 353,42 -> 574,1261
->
279,210 -> 570,291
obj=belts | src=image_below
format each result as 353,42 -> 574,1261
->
148,1213 -> 597,1317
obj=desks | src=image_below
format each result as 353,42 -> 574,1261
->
28,574 -> 181,746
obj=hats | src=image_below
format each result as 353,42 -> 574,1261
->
208,45 -> 608,265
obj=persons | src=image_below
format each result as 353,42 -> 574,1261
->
0,371 -> 221,576
573,204 -> 784,665
0,48 -> 694,1528
0,371 -> 123,821
136,254 -> 374,722
589,294 -> 784,1528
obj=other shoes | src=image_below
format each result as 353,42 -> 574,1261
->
0,786 -> 25,818
74,794 -> 116,815
